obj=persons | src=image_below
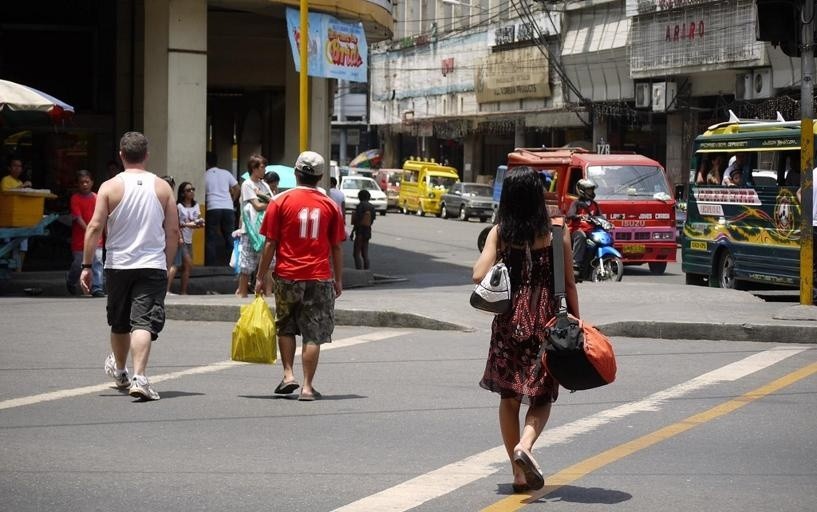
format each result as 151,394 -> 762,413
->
70,168 -> 206,296
79,131 -> 180,399
0,159 -> 34,272
350,189 -> 377,270
471,166 -> 580,494
695,151 -> 756,188
207,153 -> 347,297
797,166 -> 817,230
254,150 -> 346,399
564,177 -> 600,282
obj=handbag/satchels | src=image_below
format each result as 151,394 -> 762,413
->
470,260 -> 511,314
351,211 -> 371,225
540,312 -> 616,390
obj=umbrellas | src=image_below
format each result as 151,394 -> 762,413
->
0,80 -> 76,117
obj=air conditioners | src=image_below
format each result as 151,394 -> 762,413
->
751,64 -> 779,101
651,81 -> 680,113
733,71 -> 749,100
633,81 -> 653,110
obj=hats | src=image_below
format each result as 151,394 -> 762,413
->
297,150 -> 325,176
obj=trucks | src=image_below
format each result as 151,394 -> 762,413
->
507,148 -> 684,275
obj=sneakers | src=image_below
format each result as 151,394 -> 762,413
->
129,375 -> 159,400
104,352 -> 131,386
93,290 -> 105,297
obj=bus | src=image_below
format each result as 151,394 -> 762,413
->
681,110 -> 816,307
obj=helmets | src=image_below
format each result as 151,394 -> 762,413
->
577,178 -> 598,201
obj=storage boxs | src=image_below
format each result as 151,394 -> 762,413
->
0,187 -> 60,229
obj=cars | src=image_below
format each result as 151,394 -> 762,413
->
671,206 -> 685,242
326,155 -> 553,224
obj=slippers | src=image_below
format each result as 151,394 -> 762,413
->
275,377 -> 299,394
298,389 -> 323,401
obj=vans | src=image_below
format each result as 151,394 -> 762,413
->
750,170 -> 788,188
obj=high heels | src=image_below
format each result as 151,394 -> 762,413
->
513,451 -> 544,494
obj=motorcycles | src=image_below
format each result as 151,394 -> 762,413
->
570,200 -> 624,284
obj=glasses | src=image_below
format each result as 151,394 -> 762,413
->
185,188 -> 195,192
